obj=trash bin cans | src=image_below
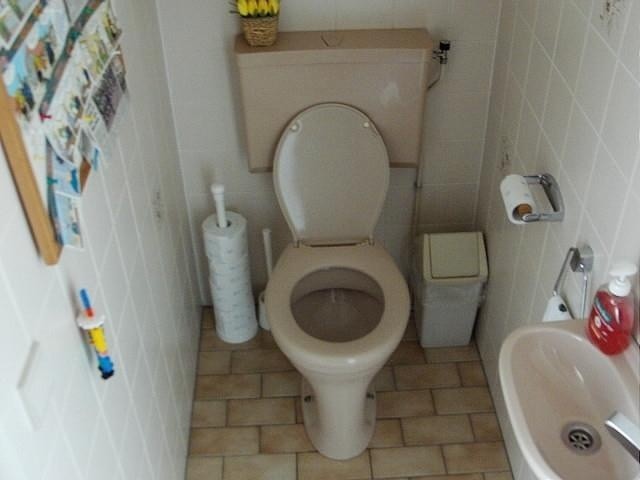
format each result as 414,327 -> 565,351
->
410,231 -> 489,349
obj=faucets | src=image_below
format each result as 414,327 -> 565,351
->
606,412 -> 640,463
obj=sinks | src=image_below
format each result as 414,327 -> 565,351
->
498,319 -> 640,480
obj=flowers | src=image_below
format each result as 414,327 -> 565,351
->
227,0 -> 281,17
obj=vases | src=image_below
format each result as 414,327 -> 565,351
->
238,15 -> 278,47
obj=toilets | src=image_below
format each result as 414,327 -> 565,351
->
235,27 -> 432,460
